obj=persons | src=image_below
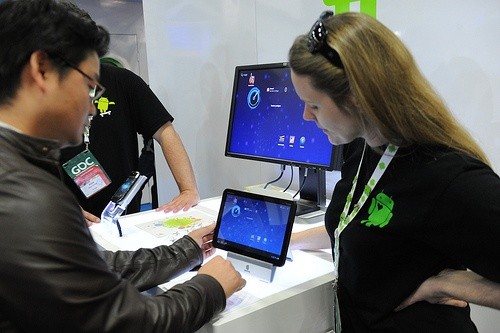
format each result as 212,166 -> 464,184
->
0,0 -> 246,333
59,63 -> 199,227
288,10 -> 500,333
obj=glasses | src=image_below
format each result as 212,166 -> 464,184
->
67,64 -> 105,102
307,10 -> 344,69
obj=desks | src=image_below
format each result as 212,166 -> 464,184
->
87,183 -> 345,333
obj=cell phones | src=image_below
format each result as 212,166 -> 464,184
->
112,171 -> 140,203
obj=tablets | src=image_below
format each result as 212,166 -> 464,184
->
214,189 -> 297,267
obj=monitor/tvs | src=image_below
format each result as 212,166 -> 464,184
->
225,62 -> 345,216
